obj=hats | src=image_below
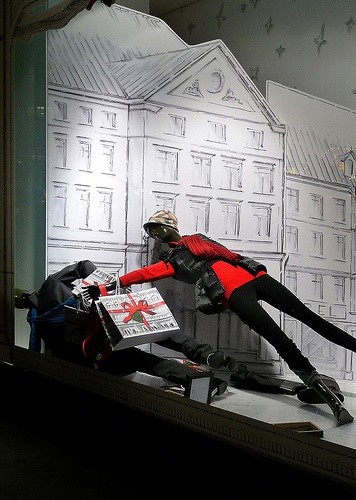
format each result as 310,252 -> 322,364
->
143,210 -> 179,242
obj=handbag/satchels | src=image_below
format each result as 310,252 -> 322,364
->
71,266 -> 133,313
88,276 -> 180,357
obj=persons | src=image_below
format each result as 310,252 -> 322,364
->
80,209 -> 356,427
7,260 -> 236,399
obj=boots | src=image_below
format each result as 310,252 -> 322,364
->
297,374 -> 345,404
